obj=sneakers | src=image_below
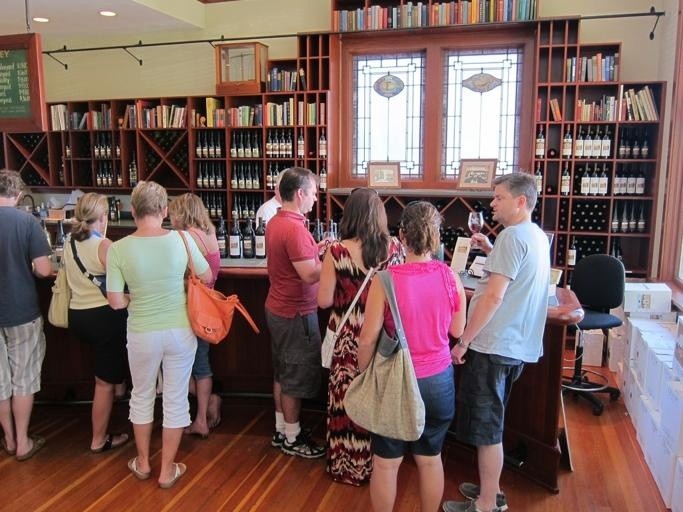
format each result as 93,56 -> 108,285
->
442,483 -> 507,512
271,427 -> 325,459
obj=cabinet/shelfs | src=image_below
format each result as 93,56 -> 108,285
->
328,0 -> 539,33
46,29 -> 330,238
532,12 -> 667,279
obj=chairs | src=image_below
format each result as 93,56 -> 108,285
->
556,250 -> 632,416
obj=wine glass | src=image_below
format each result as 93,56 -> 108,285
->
467,211 -> 483,250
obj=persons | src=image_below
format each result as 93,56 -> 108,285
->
105,180 -> 211,488
265,167 -> 334,457
167,193 -> 221,439
443,171 -> 552,512
318,187 -> 404,487
63,192 -> 128,452
255,169 -> 290,229
356,201 -> 465,511
0,169 -> 52,461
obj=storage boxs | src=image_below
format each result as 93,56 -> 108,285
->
573,327 -> 607,370
604,282 -> 683,512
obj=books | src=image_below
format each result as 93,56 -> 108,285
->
549,52 -> 659,122
536,99 -> 541,121
49,66 -> 325,131
333,0 -> 538,33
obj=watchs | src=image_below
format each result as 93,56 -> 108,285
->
457,339 -> 469,348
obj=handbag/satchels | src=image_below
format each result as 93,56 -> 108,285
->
48,267 -> 71,329
342,326 -> 425,442
320,325 -> 337,369
92,275 -> 129,298
187,275 -> 237,345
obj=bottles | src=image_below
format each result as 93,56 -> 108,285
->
556,236 -> 625,270
566,273 -> 572,290
535,123 -> 612,160
36,201 -> 48,217
193,128 -> 325,159
612,202 -> 646,235
558,200 -> 610,234
13,131 -> 188,187
53,219 -> 66,248
531,163 -> 554,228
559,161 -> 609,197
618,129 -> 652,161
437,223 -> 466,256
194,192 -> 337,259
611,164 -> 647,196
109,196 -> 117,221
193,161 -> 326,190
473,197 -> 499,229
38,219 -> 50,250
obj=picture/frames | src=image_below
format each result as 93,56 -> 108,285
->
455,157 -> 498,193
365,160 -> 402,191
0,29 -> 47,135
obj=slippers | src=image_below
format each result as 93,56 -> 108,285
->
127,457 -> 149,480
90,435 -> 126,453
1,435 -> 45,461
183,396 -> 223,439
159,463 -> 186,489
